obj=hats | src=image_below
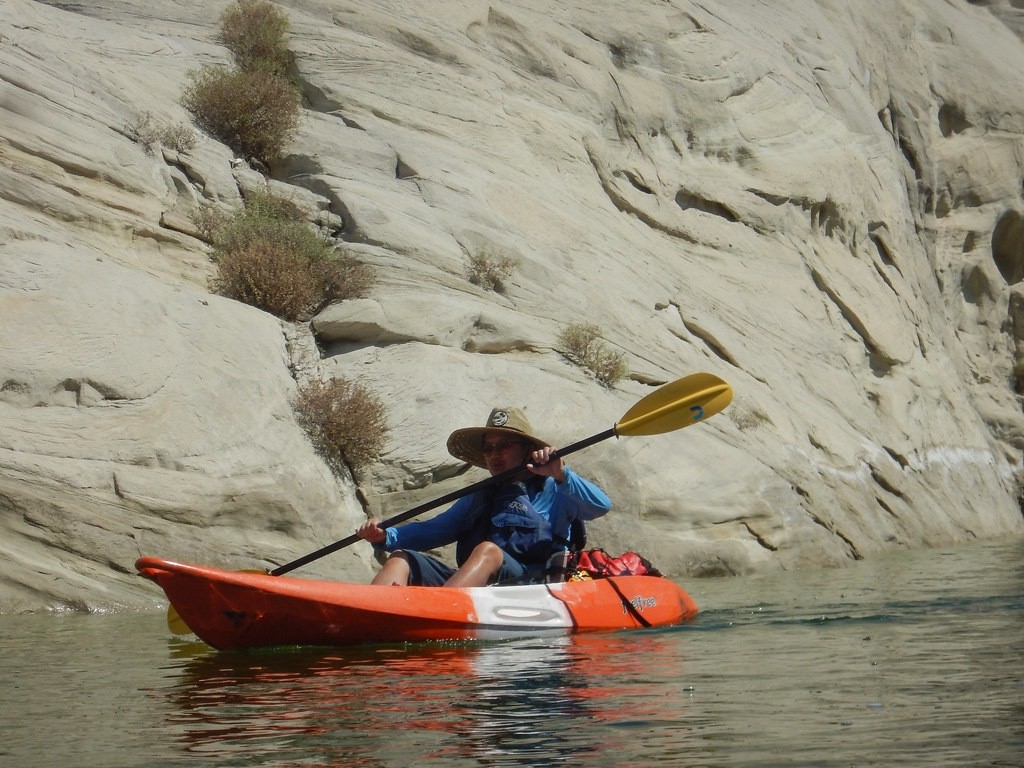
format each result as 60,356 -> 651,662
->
446,406 -> 552,469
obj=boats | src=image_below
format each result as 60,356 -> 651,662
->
133,554 -> 700,648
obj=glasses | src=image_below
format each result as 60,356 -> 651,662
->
481,441 -> 529,457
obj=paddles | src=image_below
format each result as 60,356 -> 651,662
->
166,373 -> 732,637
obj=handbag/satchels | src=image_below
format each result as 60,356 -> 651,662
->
567,547 -> 661,580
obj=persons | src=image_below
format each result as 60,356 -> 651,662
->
354,405 -> 614,587
